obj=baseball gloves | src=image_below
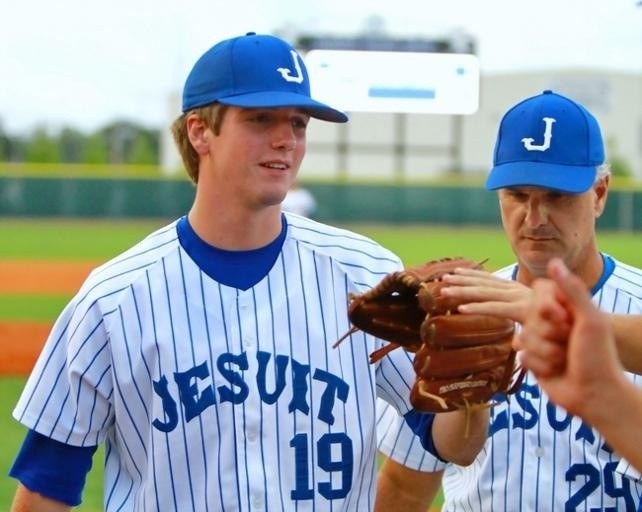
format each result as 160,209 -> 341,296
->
332,257 -> 526,438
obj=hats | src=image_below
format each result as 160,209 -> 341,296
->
182,31 -> 349,123
485,88 -> 606,195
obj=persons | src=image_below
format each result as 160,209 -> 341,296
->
3,29 -> 520,511
424,252 -> 641,472
374,82 -> 641,511
